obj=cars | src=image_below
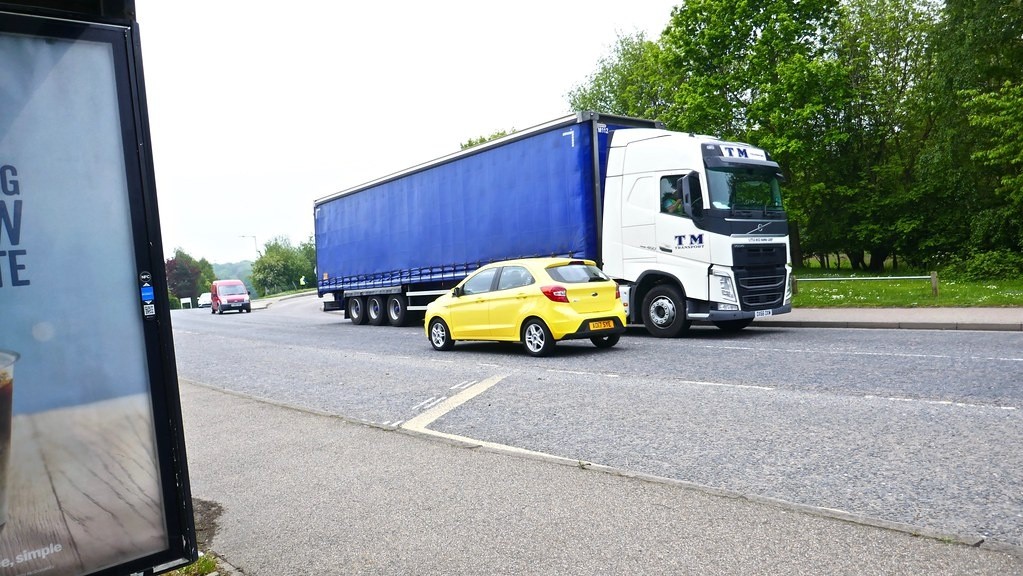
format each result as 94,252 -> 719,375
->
424,257 -> 626,357
197,293 -> 212,308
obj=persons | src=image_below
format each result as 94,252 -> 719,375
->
300,276 -> 308,289
663,188 -> 684,213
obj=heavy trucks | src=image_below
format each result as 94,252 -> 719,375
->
314,110 -> 793,338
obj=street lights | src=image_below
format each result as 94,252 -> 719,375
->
242,235 -> 258,259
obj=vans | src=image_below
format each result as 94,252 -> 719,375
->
211,280 -> 251,314
